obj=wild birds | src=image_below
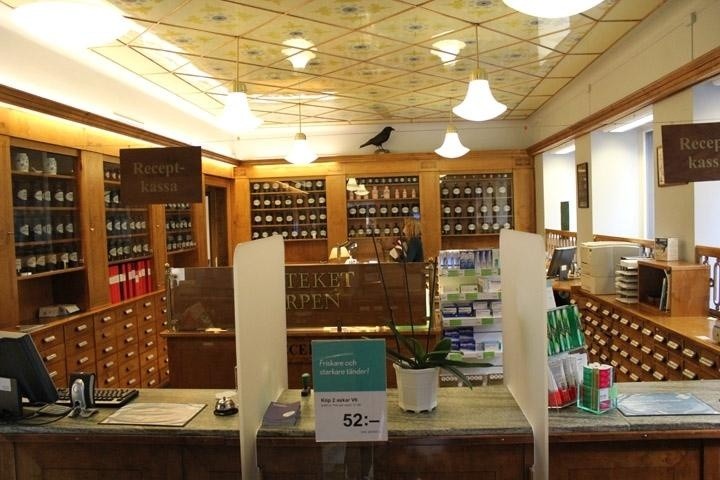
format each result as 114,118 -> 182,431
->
359,126 -> 395,152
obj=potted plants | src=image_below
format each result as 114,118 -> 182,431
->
361,222 -> 495,414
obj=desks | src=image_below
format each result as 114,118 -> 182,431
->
547,269 -> 580,290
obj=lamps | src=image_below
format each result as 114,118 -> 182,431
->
281,104 -> 320,165
214,36 -> 264,133
452,23 -> 507,122
433,114 -> 470,158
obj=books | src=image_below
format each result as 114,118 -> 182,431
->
263,400 -> 302,427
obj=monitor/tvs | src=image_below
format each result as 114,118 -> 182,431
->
547,247 -> 578,281
0,331 -> 60,424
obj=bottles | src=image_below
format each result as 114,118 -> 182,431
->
64,181 -> 74,207
254,212 -> 262,225
272,183 -> 280,192
411,203 -> 419,216
309,210 -> 317,224
394,177 -> 399,183
142,239 -> 150,256
366,225 -> 373,237
320,226 -> 327,238
503,218 -> 511,230
275,211 -> 284,224
387,178 -> 393,184
372,186 -> 378,199
481,252 -> 487,267
368,203 -> 377,218
291,228 -> 299,239
381,178 -> 386,184
30,181 -> 42,207
466,201 -> 476,217
29,214 -> 43,241
129,216 -> 135,234
359,204 -> 367,217
252,229 -> 262,240
444,203 -> 451,217
298,210 -> 307,224
285,196 -> 292,208
108,239 -> 117,261
47,158 -> 57,176
261,231 -> 270,238
502,201 -> 512,215
121,216 -> 129,235
411,189 -> 417,199
486,183 -> 494,197
131,239 -> 137,258
116,239 -> 123,260
498,182 -> 507,197
363,195 -> 368,200
479,201 -> 489,217
300,227 -> 308,239
468,219 -> 476,234
116,168 -> 121,181
281,183 -> 289,192
52,214 -> 64,240
15,249 -> 25,277
402,189 -> 408,198
392,222 -> 400,236
57,246 -> 69,270
24,248 -> 37,275
110,167 -> 117,181
402,221 -> 406,236
374,224 -> 381,237
271,228 -> 279,236
407,177 -> 412,183
34,246 -> 46,274
264,196 -> 271,208
281,227 -> 289,239
68,245 -> 78,268
252,197 -> 260,209
475,251 -> 481,268
384,224 -> 391,236
15,215 -> 30,242
253,183 -> 260,193
391,203 -> 400,217
111,189 -> 119,208
412,176 -> 417,183
310,226 -> 317,239
137,239 -> 143,257
356,179 -> 359,184
305,181 -> 313,191
285,212 -> 293,224
294,182 -> 302,190
492,219 -> 500,233
487,251 -> 493,267
122,239 -> 131,259
42,181 -> 52,207
308,194 -> 316,207
141,216 -> 147,233
167,234 -> 196,251
296,194 -> 303,207
374,178 -> 380,184
440,183 -> 450,198
474,182 -> 483,198
401,204 -> 410,216
379,203 -> 388,217
263,183 -> 271,192
356,194 -> 361,201
454,202 -> 463,217
368,179 -> 373,184
104,167 -> 112,180
113,216 -> 121,235
361,179 -> 366,184
104,187 -> 111,209
13,180 -> 29,207
357,225 -> 364,237
452,184 -> 461,198
265,212 -> 273,225
492,201 -> 502,216
348,191 -> 354,201
348,225 -> 356,237
481,220 -> 490,233
319,209 -> 326,223
394,189 -> 400,199
399,177 -> 405,183
383,186 -> 391,200
349,204 -> 357,218
45,246 -> 57,271
42,214 -> 53,241
274,195 -> 282,208
454,220 -> 463,234
136,216 -> 142,233
464,182 -> 472,198
15,152 -> 29,172
106,216 -> 113,236
441,220 -> 451,235
315,181 -> 324,190
318,194 -> 326,207
51,181 -> 65,207
63,214 -> 74,238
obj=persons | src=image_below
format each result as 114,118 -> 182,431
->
398,222 -> 422,262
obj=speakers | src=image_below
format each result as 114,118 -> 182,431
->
68,373 -> 98,417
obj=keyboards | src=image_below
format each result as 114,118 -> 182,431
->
56,387 -> 139,407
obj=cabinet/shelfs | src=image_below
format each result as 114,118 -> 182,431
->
0,83 -> 208,389
569,282 -> 720,382
437,248 -> 503,386
232,149 -> 535,263
615,259 -> 640,305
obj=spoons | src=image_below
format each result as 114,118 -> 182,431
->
31,166 -> 43,174
